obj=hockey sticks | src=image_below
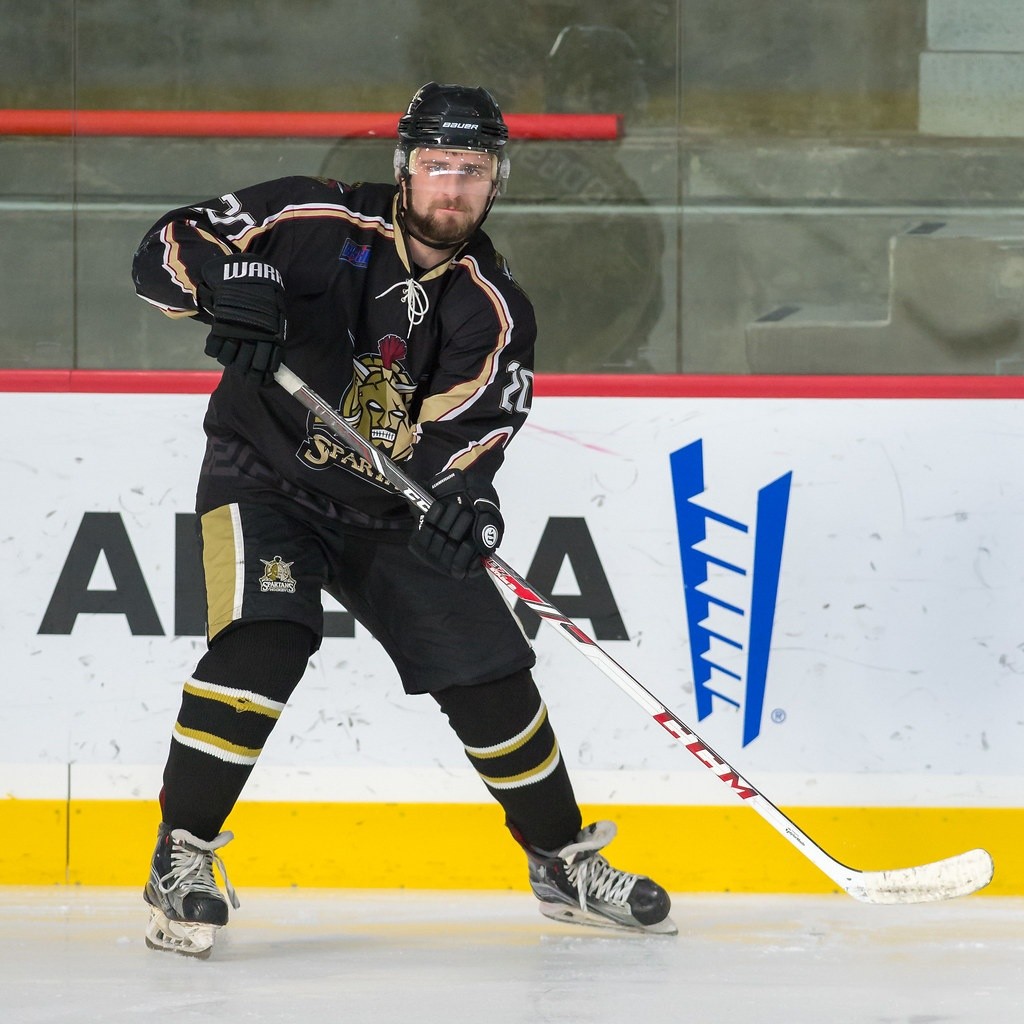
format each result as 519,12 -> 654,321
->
270,364 -> 996,905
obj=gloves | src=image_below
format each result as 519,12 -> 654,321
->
205,258 -> 285,375
408,468 -> 504,583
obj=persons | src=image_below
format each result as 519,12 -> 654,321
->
318,24 -> 668,373
134,82 -> 679,960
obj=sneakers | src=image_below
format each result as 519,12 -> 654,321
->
142,822 -> 241,954
514,819 -> 679,941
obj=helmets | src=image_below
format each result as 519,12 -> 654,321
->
392,80 -> 509,153
